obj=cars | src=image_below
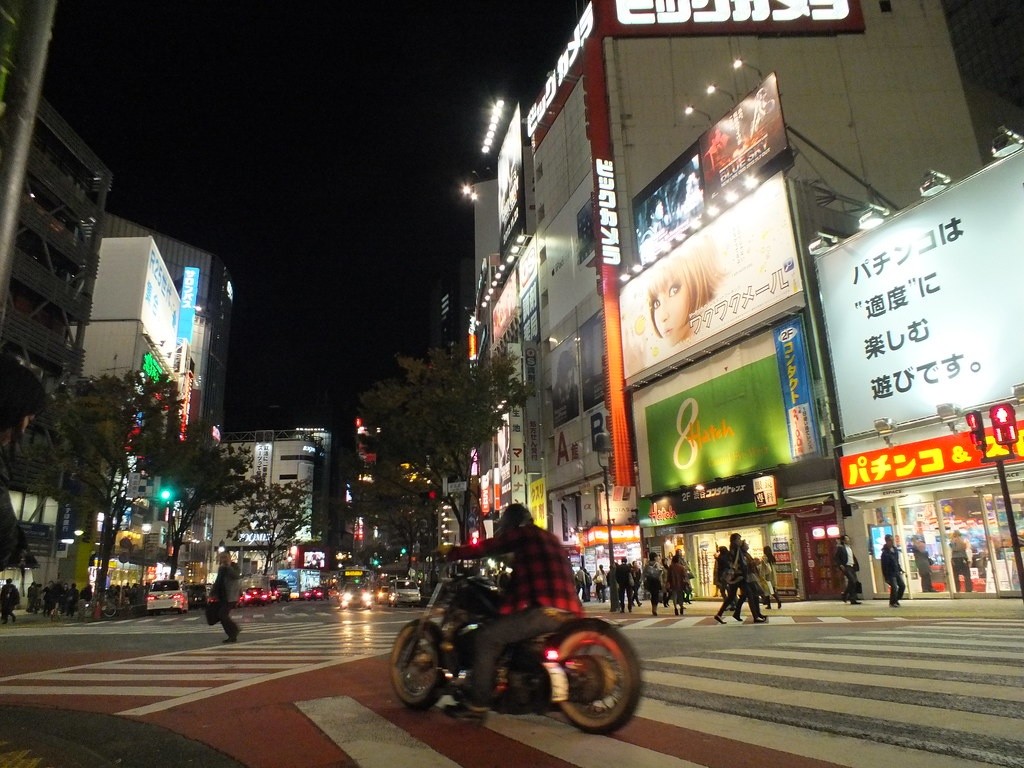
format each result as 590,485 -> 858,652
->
188,583 -> 213,609
377,579 -> 422,607
235,580 -> 339,607
147,579 -> 189,616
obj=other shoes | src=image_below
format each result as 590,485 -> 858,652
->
764,606 -> 771,609
729,603 -> 735,611
753,618 -> 764,623
732,614 -> 743,622
619,601 -> 692,616
841,592 -> 847,604
221,631 -> 235,643
851,601 -> 861,604
891,601 -> 900,607
714,615 -> 727,624
442,701 -> 489,720
778,601 -> 781,608
1,615 -> 16,625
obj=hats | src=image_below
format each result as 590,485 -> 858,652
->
503,503 -> 532,532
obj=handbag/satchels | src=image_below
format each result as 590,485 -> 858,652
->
629,573 -> 634,587
593,570 -> 603,584
215,580 -> 226,603
928,557 -> 934,565
687,571 -> 695,579
725,563 -> 743,584
856,581 -> 863,594
204,602 -> 222,627
763,580 -> 775,596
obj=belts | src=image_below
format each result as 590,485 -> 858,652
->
846,565 -> 854,567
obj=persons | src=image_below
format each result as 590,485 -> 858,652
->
615,548 -> 693,616
26,581 -> 92,617
441,503 -> 583,727
209,552 -> 240,644
307,554 -> 320,568
645,237 -> 726,350
914,540 -> 936,592
0,578 -> 20,624
105,582 -> 150,599
641,166 -> 703,244
834,535 -> 860,605
713,533 -> 782,624
880,534 -> 906,608
574,565 -> 611,603
949,530 -> 973,592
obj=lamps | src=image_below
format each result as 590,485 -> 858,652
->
874,382 -> 1024,441
809,126 -> 1024,256
481,235 -> 533,309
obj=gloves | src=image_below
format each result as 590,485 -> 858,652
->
439,544 -> 458,559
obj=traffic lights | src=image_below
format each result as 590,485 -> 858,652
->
470,528 -> 480,544
162,490 -> 172,499
400,548 -> 407,555
966,410 -> 987,451
989,403 -> 1019,445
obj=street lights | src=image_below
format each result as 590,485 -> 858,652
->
595,432 -> 621,613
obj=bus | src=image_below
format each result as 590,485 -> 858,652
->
339,570 -> 377,609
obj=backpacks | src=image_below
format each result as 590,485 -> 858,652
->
585,572 -> 592,587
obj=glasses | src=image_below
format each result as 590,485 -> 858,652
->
717,550 -> 720,553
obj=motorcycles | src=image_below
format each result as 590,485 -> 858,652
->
390,556 -> 642,735
